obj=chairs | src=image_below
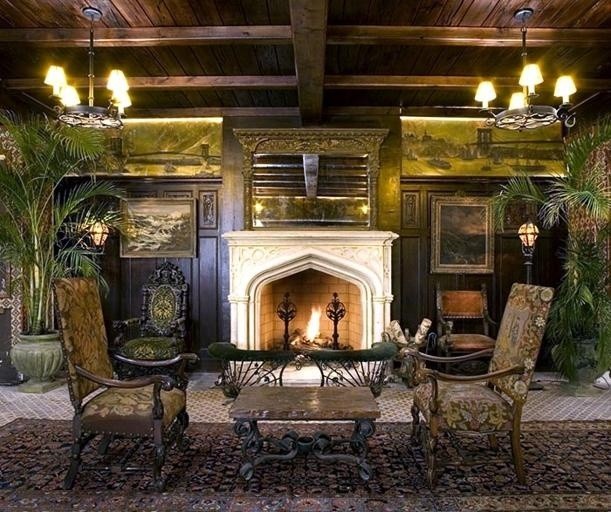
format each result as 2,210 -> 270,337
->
106,261 -> 203,388
403,281 -> 557,495
432,280 -> 500,376
43,266 -> 194,496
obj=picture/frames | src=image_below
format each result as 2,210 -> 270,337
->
427,193 -> 498,277
116,195 -> 202,262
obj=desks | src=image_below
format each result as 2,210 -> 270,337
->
226,384 -> 383,494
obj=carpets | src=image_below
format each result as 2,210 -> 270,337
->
0,415 -> 611,511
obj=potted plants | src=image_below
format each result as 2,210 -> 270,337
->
0,104 -> 140,396
490,115 -> 611,386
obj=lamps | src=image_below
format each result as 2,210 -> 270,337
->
519,215 -> 541,286
40,5 -> 135,133
78,212 -> 113,264
472,5 -> 579,135
